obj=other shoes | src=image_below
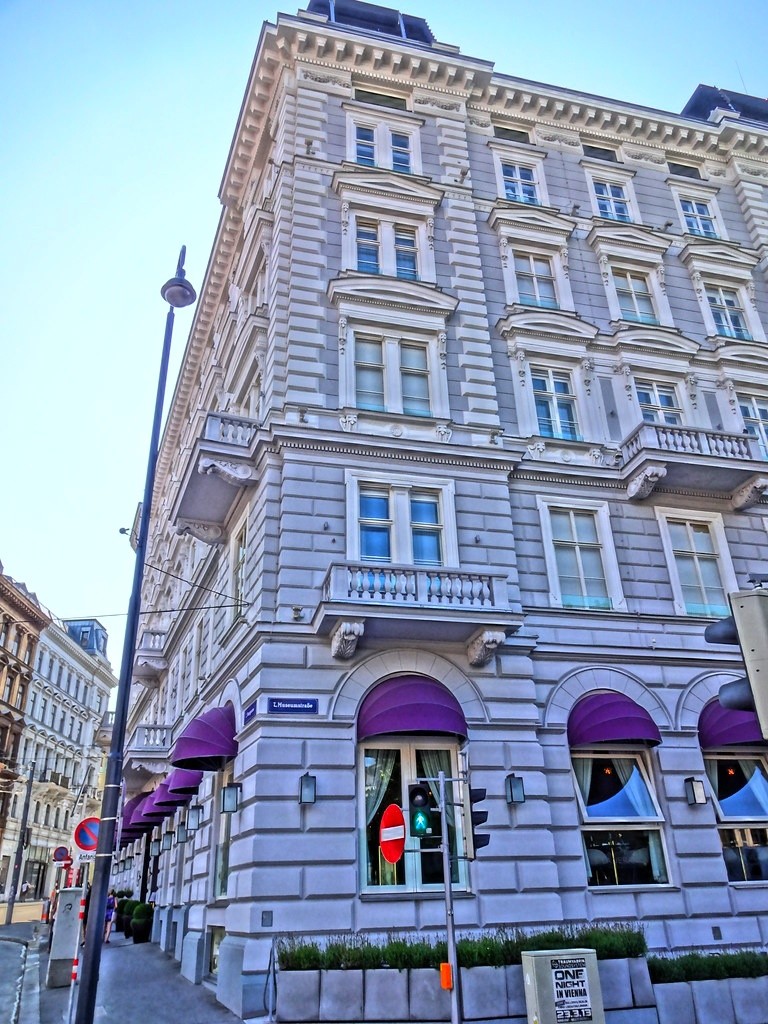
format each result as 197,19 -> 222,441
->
24,900 -> 25,902
105,940 -> 110,944
21,901 -> 23,903
80,941 -> 85,947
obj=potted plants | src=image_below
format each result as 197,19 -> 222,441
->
581,918 -> 634,1012
274,933 -> 321,1022
318,932 -> 365,1023
645,955 -> 696,1024
122,900 -> 141,939
116,899 -> 127,932
723,949 -> 768,1024
408,936 -> 453,1022
624,921 -> 657,1008
688,951 -> 736,1024
131,903 -> 154,944
361,933 -> 410,1022
500,927 -> 527,1017
456,928 -> 509,1021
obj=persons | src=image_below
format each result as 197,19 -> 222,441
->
80,879 -> 93,946
21,881 -> 30,903
105,889 -> 118,944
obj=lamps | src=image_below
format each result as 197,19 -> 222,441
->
119,860 -> 125,873
112,863 -> 119,875
684,776 -> 707,806
220,782 -> 243,813
124,855 -> 134,870
299,772 -> 317,805
162,830 -> 176,851
176,821 -> 187,843
185,805 -> 205,830
504,772 -> 526,805
151,839 -> 162,857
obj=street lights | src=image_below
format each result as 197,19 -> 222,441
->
74,242 -> 198,1024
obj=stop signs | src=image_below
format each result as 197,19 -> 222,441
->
379,804 -> 406,864
61,856 -> 74,869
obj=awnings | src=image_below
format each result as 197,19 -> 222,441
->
119,707 -> 239,850
698,698 -> 768,748
567,693 -> 663,749
358,675 -> 469,742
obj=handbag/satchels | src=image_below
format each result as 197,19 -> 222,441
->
107,904 -> 114,909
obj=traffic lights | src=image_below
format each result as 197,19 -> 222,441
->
408,784 -> 433,837
463,783 -> 491,862
